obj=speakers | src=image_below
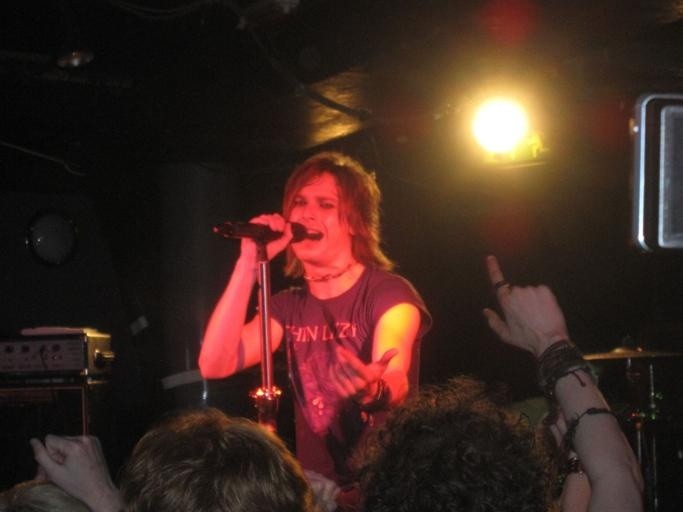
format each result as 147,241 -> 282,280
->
0,380 -> 120,492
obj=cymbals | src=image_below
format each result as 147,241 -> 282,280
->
582,345 -> 683,361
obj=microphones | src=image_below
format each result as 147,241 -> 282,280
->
214,221 -> 307,243
